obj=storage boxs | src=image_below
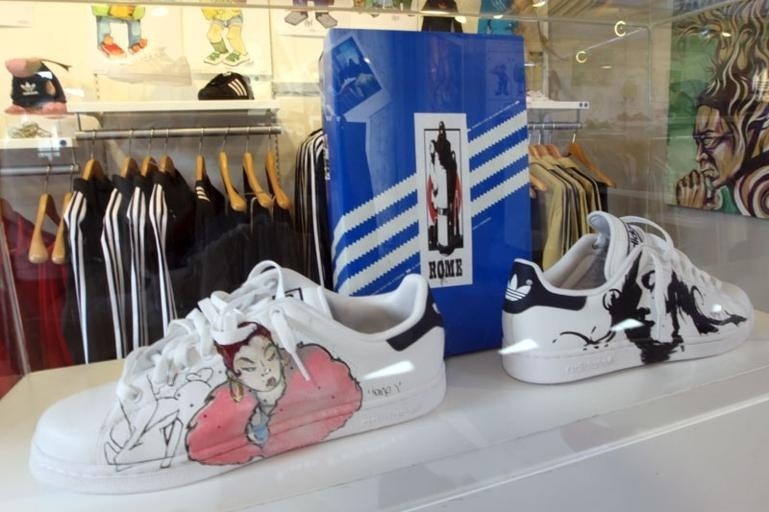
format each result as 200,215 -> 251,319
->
317,28 -> 532,359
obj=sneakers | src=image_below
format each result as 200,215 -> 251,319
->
28,265 -> 446,493
502,211 -> 752,385
199,73 -> 253,99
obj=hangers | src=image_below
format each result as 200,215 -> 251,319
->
526,121 -> 615,200
28,126 -> 292,266
